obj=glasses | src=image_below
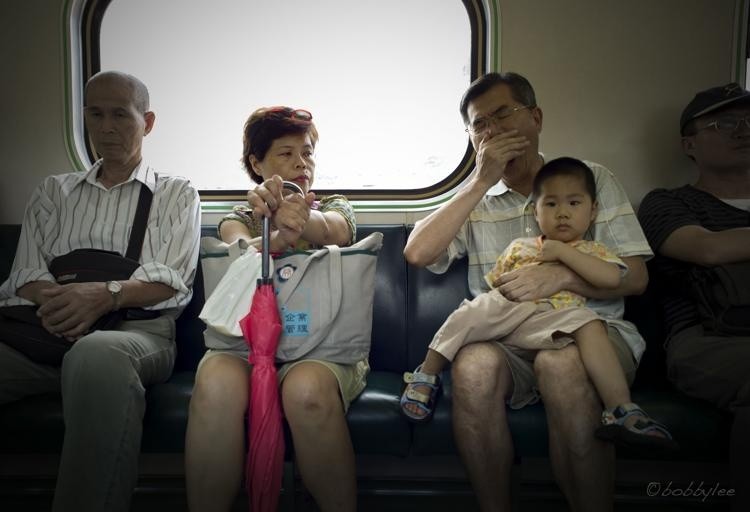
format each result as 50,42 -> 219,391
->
261,106 -> 313,125
464,105 -> 529,136
689,114 -> 750,136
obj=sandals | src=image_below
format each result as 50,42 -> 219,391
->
598,401 -> 672,445
399,363 -> 441,422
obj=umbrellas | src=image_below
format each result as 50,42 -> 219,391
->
241,182 -> 305,510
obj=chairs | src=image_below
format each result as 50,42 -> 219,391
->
0,225 -> 228,445
244,223 -> 404,467
404,225 -> 696,457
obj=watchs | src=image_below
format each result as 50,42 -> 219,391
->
106,280 -> 122,315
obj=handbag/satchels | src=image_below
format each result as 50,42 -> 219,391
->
199,231 -> 383,362
2,171 -> 160,368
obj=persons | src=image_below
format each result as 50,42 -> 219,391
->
0,73 -> 201,512
404,71 -> 653,510
634,87 -> 748,496
401,157 -> 674,452
185,110 -> 373,512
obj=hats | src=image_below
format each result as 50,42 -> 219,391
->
680,82 -> 748,130
